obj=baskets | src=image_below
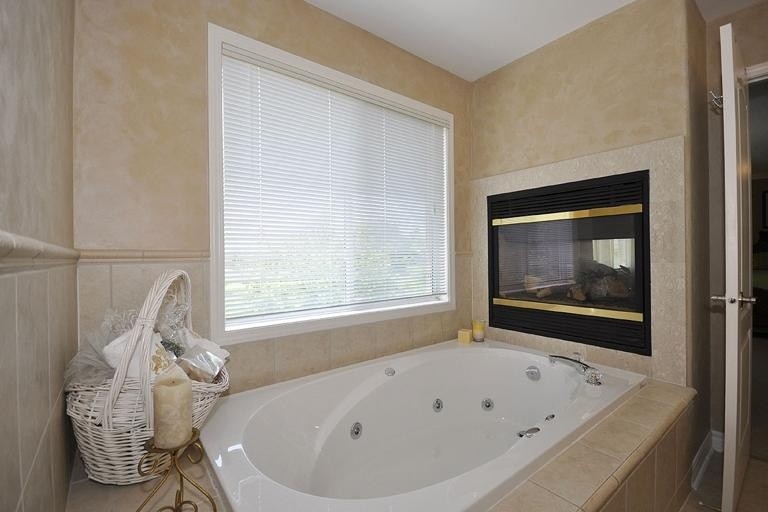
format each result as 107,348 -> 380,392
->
64,268 -> 230,484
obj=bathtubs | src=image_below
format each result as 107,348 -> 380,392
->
194,337 -> 646,511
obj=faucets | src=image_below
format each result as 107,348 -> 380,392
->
548,354 -> 600,385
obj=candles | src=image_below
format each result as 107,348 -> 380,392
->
153,378 -> 193,449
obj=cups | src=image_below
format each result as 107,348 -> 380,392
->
455,319 -> 486,345
